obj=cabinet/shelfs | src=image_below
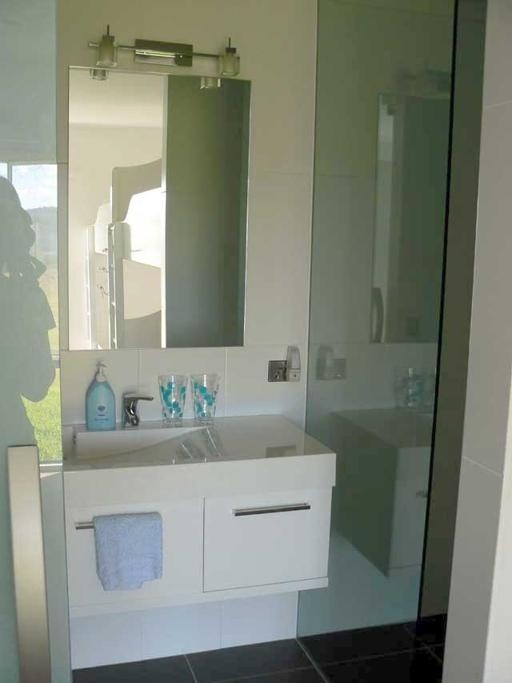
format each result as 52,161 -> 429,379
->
202,488 -> 333,592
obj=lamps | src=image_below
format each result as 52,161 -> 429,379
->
87,24 -> 241,75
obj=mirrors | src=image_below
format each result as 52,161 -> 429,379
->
66,64 -> 253,348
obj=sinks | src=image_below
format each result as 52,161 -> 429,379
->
63,426 -> 227,470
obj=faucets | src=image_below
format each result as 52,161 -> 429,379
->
122,391 -> 154,429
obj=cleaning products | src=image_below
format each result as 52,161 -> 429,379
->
84,361 -> 116,431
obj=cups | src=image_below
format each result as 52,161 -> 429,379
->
190,371 -> 226,420
397,367 -> 435,411
159,373 -> 188,422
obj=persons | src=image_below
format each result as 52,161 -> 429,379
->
0,177 -> 58,452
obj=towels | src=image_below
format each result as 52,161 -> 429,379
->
93,510 -> 164,594
